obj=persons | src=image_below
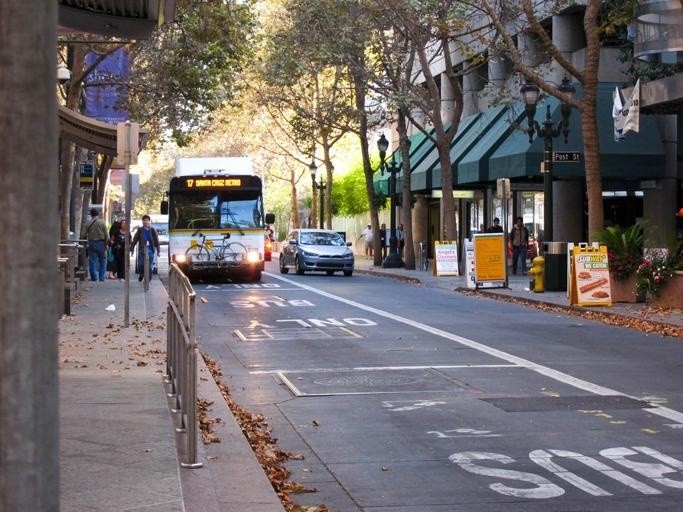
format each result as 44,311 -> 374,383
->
130,214 -> 161,282
508,215 -> 529,277
487,216 -> 503,232
106,220 -> 121,280
109,218 -> 134,284
356,223 -> 374,261
379,223 -> 386,262
394,222 -> 404,256
78,208 -> 108,282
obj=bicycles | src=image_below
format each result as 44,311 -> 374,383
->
185,230 -> 248,262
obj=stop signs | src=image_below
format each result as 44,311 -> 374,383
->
111,170 -> 125,186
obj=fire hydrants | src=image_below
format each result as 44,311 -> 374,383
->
528,254 -> 545,293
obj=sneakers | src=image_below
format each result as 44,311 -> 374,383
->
108,275 -> 115,279
365,255 -> 372,260
512,271 -> 528,275
139,274 -> 144,281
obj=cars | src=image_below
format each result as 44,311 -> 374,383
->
279,229 -> 354,275
264,231 -> 273,261
156,230 -> 169,245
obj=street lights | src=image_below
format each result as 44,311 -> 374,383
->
520,73 -> 576,242
310,159 -> 326,228
377,134 -> 402,268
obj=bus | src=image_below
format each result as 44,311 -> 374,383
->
160,158 -> 275,282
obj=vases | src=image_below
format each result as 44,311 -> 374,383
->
608,270 -> 646,303
650,270 -> 682,309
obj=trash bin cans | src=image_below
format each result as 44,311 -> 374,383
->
543,242 -> 567,292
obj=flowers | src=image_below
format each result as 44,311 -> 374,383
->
607,251 -> 642,281
632,248 -> 674,298
